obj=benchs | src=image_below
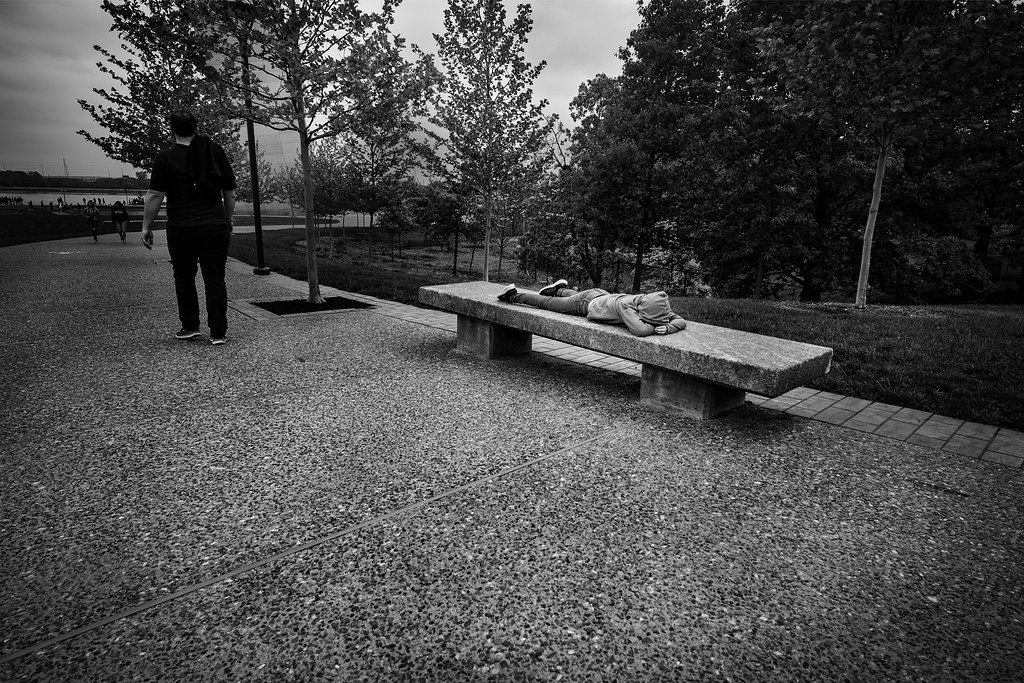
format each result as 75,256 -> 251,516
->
420,278 -> 833,420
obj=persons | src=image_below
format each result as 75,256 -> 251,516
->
0,196 -> 144,212
110,201 -> 130,243
141,110 -> 236,344
82,200 -> 103,243
497,279 -> 686,336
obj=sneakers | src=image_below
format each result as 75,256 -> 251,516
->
497,283 -> 517,302
539,279 -> 568,296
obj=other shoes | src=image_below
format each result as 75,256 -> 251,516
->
210,336 -> 228,345
175,328 -> 201,339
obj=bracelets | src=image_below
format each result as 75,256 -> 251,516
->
664,325 -> 668,335
227,220 -> 235,222
